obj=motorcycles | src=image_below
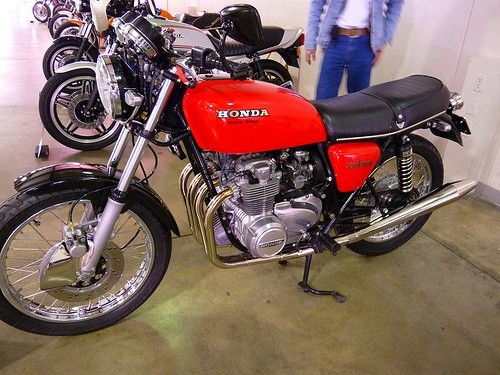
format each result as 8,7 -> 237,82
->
0,5 -> 472,336
33,0 -> 305,151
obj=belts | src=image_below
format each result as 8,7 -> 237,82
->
333,27 -> 369,37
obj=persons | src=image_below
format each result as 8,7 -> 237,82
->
305,0 -> 404,101
